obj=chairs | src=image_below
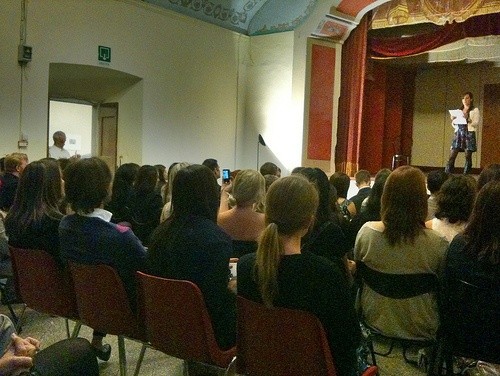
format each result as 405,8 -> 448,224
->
391,135 -> 413,170
0,244 -> 500,376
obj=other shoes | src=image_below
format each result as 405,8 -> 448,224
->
419,354 -> 442,376
90,344 -> 111,362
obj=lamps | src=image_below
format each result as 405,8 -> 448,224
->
255,133 -> 267,173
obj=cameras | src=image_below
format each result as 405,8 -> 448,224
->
221,169 -> 230,182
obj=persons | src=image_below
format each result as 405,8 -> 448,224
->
0,154 -> 500,376
445,92 -> 480,175
48,131 -> 80,161
